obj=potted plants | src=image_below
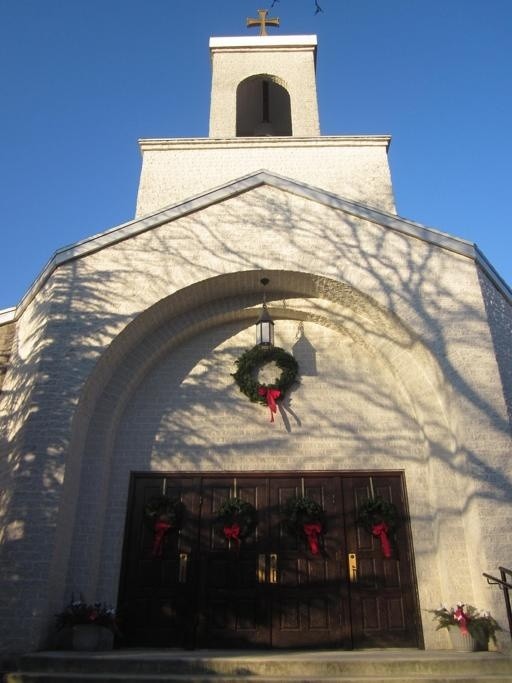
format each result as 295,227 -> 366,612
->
429,604 -> 497,652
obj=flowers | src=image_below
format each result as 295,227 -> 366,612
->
68,600 -> 123,637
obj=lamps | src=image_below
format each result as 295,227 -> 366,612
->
256,278 -> 274,346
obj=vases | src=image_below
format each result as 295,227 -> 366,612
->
72,625 -> 114,651
